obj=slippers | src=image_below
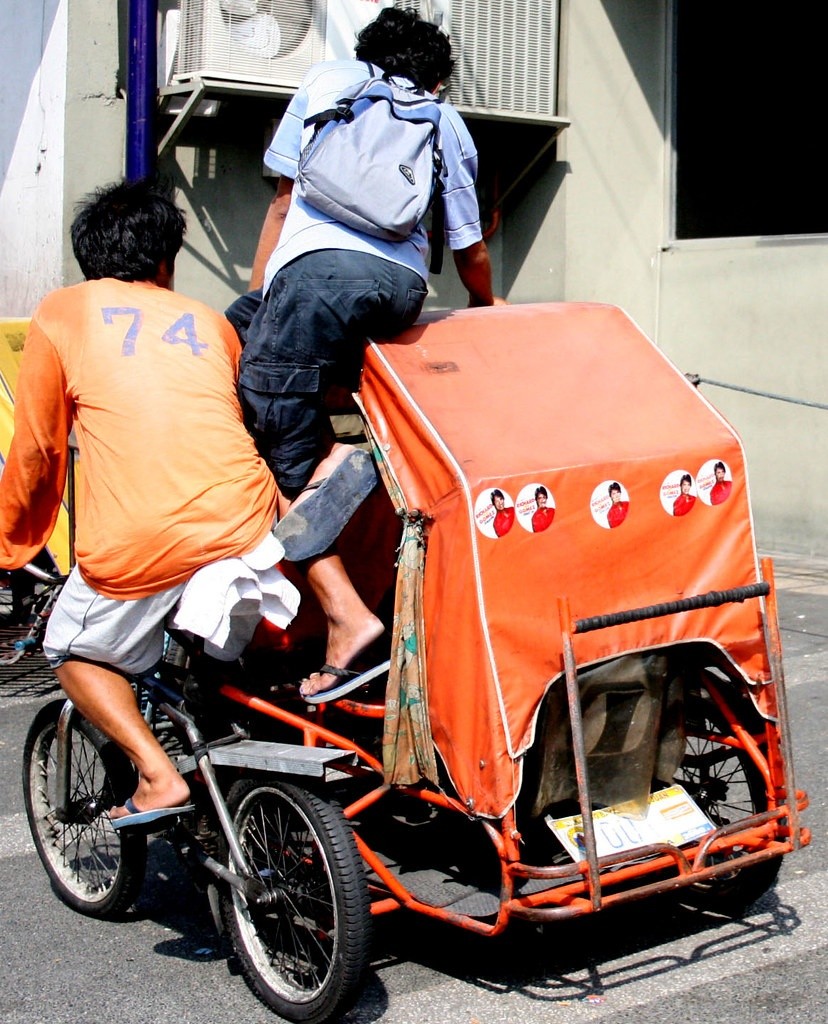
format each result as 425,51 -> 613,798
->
299,633 -> 393,704
271,448 -> 381,563
108,797 -> 197,830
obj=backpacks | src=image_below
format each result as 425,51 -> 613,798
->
294,62 -> 452,274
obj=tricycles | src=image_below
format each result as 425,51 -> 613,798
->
0,299 -> 811,1024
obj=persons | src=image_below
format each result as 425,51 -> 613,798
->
227,7 -> 508,703
491,490 -> 514,537
608,482 -> 628,529
0,179 -> 277,830
711,461 -> 732,505
674,474 -> 695,517
532,487 -> 555,531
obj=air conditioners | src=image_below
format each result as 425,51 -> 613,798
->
393,0 -> 559,118
173,0 -> 394,88
